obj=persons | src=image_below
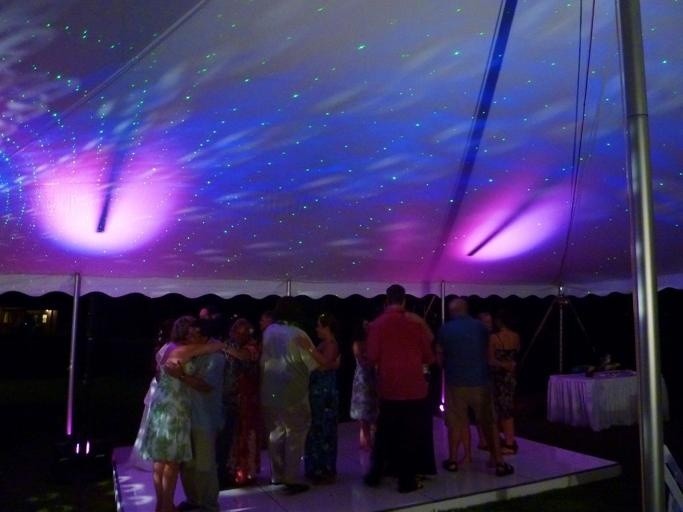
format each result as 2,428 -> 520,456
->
349,285 -> 434,495
435,298 -> 519,474
134,304 -> 340,511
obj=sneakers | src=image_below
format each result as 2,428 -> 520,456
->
271,479 -> 310,495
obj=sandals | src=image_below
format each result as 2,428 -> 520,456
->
443,440 -> 518,476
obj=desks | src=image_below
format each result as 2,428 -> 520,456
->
544,367 -> 671,432
